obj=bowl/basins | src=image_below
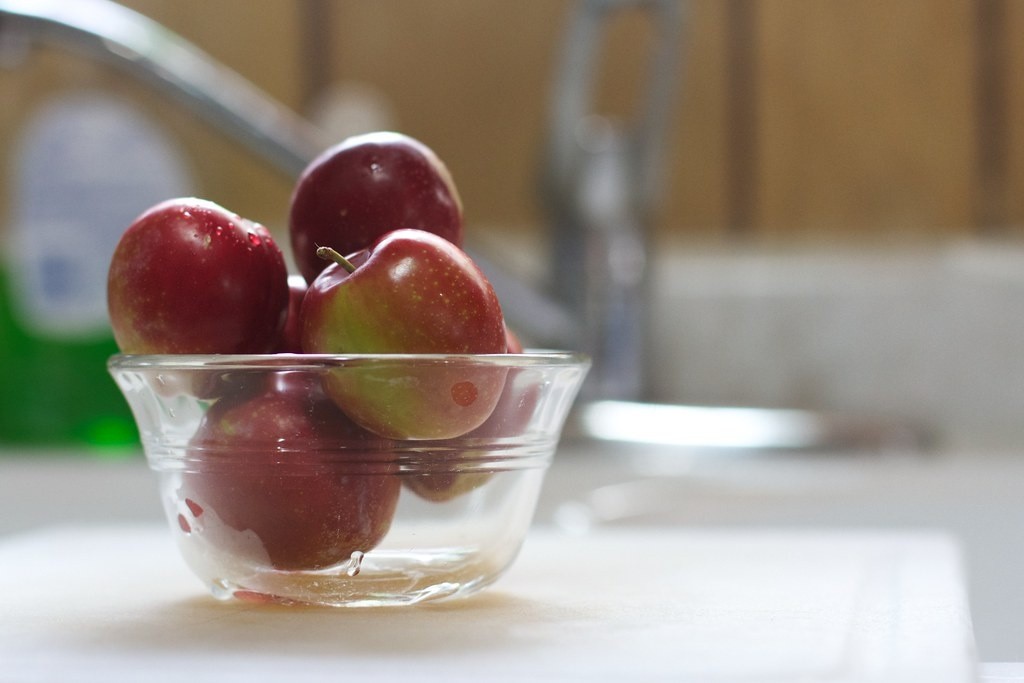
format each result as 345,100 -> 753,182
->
108,354 -> 591,609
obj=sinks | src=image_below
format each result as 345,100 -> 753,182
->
558,406 -> 945,453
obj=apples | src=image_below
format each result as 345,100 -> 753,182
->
298,226 -> 507,439
184,370 -> 399,571
274,275 -> 311,355
289,131 -> 461,285
398,322 -> 538,500
108,195 -> 287,397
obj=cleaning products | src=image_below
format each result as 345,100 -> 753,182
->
0,32 -> 204,451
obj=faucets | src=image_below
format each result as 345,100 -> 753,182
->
0,4 -> 682,414
